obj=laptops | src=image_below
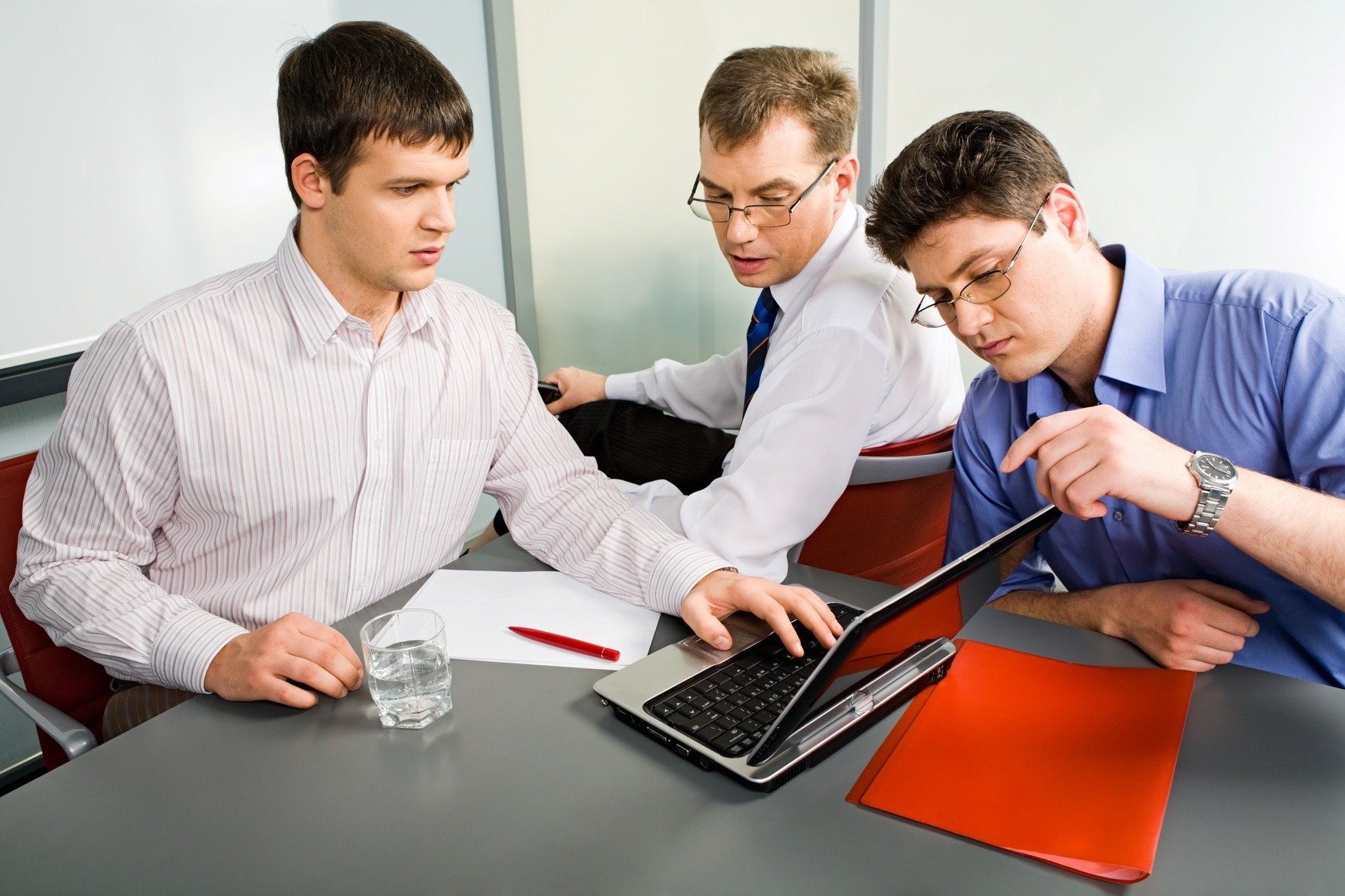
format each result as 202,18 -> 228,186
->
589,501 -> 1064,791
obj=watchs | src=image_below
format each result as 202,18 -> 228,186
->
1175,449 -> 1238,536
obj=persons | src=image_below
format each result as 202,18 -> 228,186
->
458,47 -> 964,585
7,23 -> 844,746
866,109 -> 1345,693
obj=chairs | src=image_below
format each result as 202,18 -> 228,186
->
0,451 -> 134,769
791,427 -> 954,589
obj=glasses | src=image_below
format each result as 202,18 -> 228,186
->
687,159 -> 838,229
910,193 -> 1051,328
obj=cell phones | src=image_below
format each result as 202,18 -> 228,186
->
537,381 -> 562,405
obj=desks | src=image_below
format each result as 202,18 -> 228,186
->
0,532 -> 1345,896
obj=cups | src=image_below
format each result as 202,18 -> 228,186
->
360,609 -> 454,730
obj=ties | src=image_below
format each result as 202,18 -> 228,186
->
742,287 -> 780,422
